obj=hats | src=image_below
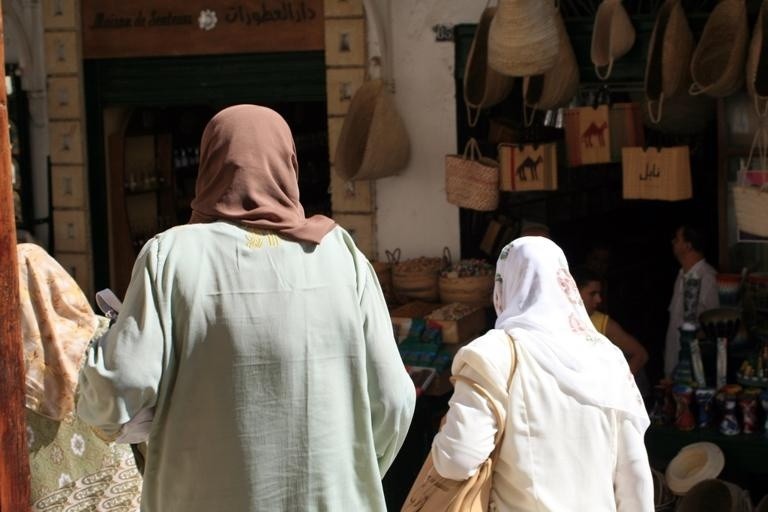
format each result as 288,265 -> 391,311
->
665,442 -> 725,497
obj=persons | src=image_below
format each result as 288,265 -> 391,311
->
432,235 -> 654,512
570,268 -> 647,379
662,228 -> 727,383
73,103 -> 417,511
14,240 -> 142,512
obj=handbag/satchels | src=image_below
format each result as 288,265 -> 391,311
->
445,139 -> 500,211
732,128 -> 767,238
500,132 -> 558,193
94,288 -> 155,446
621,129 -> 693,201
561,88 -> 646,169
401,331 -> 518,512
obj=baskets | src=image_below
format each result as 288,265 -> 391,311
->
463,0 -> 767,127
390,248 -> 439,304
328,56 -> 410,194
368,250 -> 389,301
